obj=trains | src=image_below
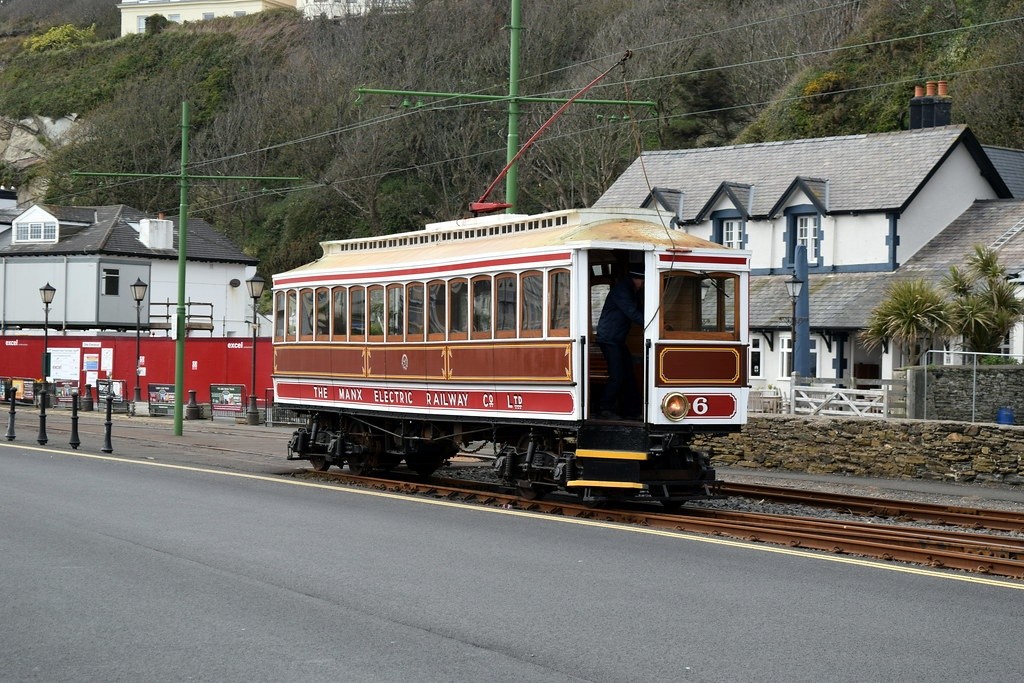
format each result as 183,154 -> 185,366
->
271,209 -> 749,510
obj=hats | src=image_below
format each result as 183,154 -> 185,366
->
629,263 -> 646,280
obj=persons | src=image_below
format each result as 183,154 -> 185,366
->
596,263 -> 645,420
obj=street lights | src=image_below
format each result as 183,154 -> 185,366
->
130,276 -> 148,415
39,282 -> 57,407
245,271 -> 267,426
785,269 -> 803,376
